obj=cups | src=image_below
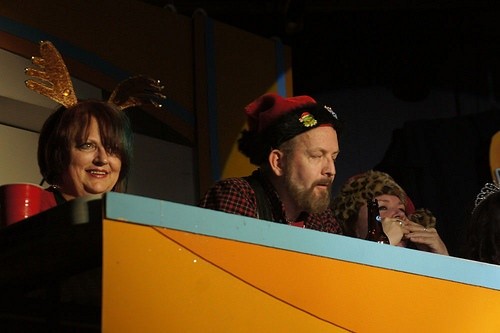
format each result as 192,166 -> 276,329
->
1,182 -> 44,227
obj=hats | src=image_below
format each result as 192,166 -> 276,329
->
331,170 -> 408,231
238,92 -> 341,165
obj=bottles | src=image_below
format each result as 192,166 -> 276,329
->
365,198 -> 391,244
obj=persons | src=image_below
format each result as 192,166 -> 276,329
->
332,171 -> 449,256
37,99 -> 136,212
203,94 -> 344,235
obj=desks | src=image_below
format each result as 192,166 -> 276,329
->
0,192 -> 500,333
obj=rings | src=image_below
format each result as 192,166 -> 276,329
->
399,221 -> 402,227
423,227 -> 427,232
